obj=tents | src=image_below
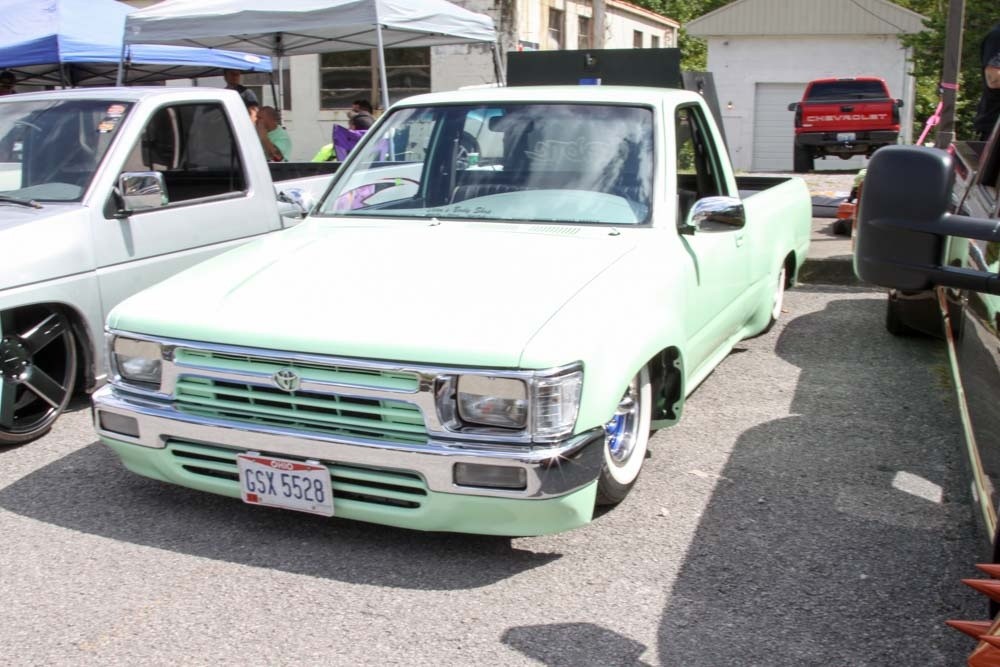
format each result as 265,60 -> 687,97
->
117,1 -> 508,112
0,0 -> 274,87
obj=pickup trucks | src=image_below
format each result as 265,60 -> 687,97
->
850,114 -> 1000,667
787,77 -> 904,173
0,84 -> 428,449
88,81 -> 812,536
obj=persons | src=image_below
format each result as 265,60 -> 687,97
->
309,99 -> 387,163
224,83 -> 293,163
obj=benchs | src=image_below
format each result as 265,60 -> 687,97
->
46,165 -> 237,204
444,172 -> 696,227
